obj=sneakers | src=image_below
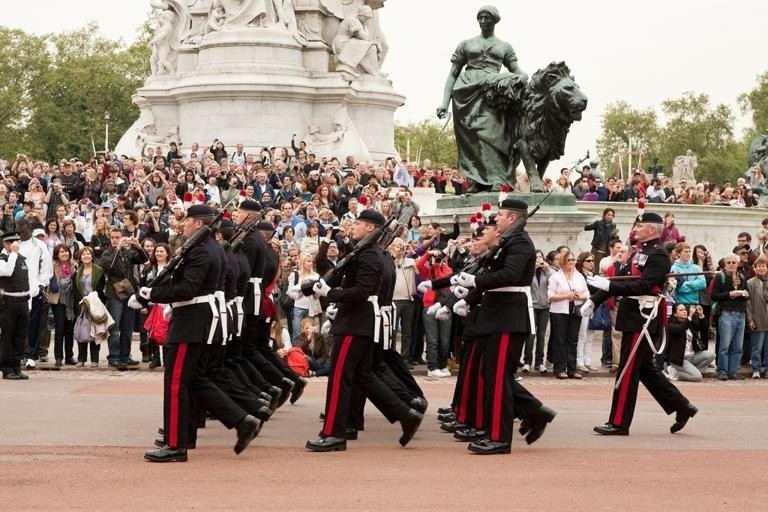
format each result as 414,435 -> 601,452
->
544,358 -> 554,373
39,355 -> 48,363
576,366 -> 589,373
568,369 -> 583,380
667,365 -> 679,381
64,357 -> 78,365
124,358 -> 139,366
602,360 -> 612,368
306,369 -> 316,377
586,365 -> 598,371
535,364 -> 549,373
554,368 -> 567,380
522,363 -> 531,373
605,363 -> 618,372
751,370 -> 761,379
75,360 -> 84,367
25,358 -> 37,368
142,352 -> 152,362
107,357 -> 130,369
54,358 -> 62,367
764,369 -> 768,379
89,361 -> 98,368
149,357 -> 161,369
403,357 -> 460,379
718,372 -> 730,380
727,374 -> 746,380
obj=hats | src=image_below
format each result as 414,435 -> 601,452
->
497,199 -> 529,212
193,178 -> 206,185
185,204 -> 216,218
472,227 -> 486,237
99,201 -> 114,210
239,200 -> 262,213
256,222 -> 277,233
355,209 -> 386,226
733,244 -> 751,254
215,219 -> 234,231
0,231 -> 23,243
32,228 -> 47,238
96,166 -> 104,174
636,213 -> 664,224
22,199 -> 35,209
484,215 -> 499,227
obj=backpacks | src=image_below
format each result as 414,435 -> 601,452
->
711,271 -> 746,314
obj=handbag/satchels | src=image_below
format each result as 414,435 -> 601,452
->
279,270 -> 300,313
143,302 -> 170,346
588,301 -> 613,332
568,299 -> 584,319
72,305 -> 100,344
112,278 -> 136,303
429,266 -> 447,304
688,324 -> 709,351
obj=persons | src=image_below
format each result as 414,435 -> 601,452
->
395,190 -> 421,223
574,164 -> 595,183
340,214 -> 356,233
547,252 -> 590,378
330,157 -> 340,168
110,163 -> 129,187
307,169 -> 322,192
435,169 -> 462,196
99,202 -> 124,230
41,178 -> 69,220
119,155 -> 129,165
0,184 -> 7,206
205,162 -> 218,177
93,207 -> 104,226
412,162 -> 418,173
41,162 -> 52,183
584,207 -> 618,276
671,148 -> 698,182
294,317 -> 332,377
408,214 -> 421,241
380,202 -> 391,217
145,170 -> 171,204
148,156 -> 170,180
277,202 -> 302,226
245,154 -> 256,170
624,179 -> 642,202
451,237 -> 468,261
751,189 -> 763,205
228,143 -> 247,166
646,180 -> 663,202
732,244 -> 756,279
306,192 -> 326,209
112,195 -> 130,224
360,195 -> 376,211
662,376 -> 664,377
555,176 -> 572,195
99,181 -> 118,200
573,177 -> 589,197
62,220 -> 86,245
358,164 -> 370,186
206,2 -> 224,34
389,242 -> 419,369
299,180 -> 313,201
575,251 -> 613,369
183,169 -> 197,191
0,160 -> 10,177
692,245 -> 715,355
454,198 -> 559,454
97,155 -> 106,166
320,156 -> 330,169
3,166 -> 17,183
263,207 -> 277,220
385,243 -> 400,262
94,208 -> 109,224
714,252 -> 748,381
753,242 -> 768,263
81,169 -> 101,197
72,247 -> 106,368
330,5 -> 389,80
275,224 -> 298,255
295,150 -> 309,165
737,232 -> 752,247
203,175 -> 220,203
74,160 -> 83,174
32,168 -> 41,178
192,179 -> 209,201
60,164 -> 76,195
392,236 -> 404,246
416,169 -> 433,186
758,218 -> 768,240
126,204 -> 260,462
426,213 -> 460,253
381,170 -> 398,187
287,252 -> 325,339
120,160 -> 132,174
4,175 -> 17,191
44,218 -> 65,245
185,142 -> 202,161
33,230 -> 47,247
522,247 -> 554,374
211,216 -> 272,431
449,166 -> 458,181
136,235 -> 156,366
599,247 -> 632,367
0,231 -> 31,380
251,161 -> 264,175
268,147 -> 277,158
263,164 -> 273,179
599,239 -> 622,280
746,256 -> 768,380
146,205 -> 164,233
616,179 -> 626,192
152,243 -> 170,272
602,179 -> 610,187
302,205 -> 318,223
230,200 -> 294,410
338,173 -> 362,202
147,0 -> 176,76
166,177 -> 175,191
194,146 -> 196,147
48,244 -> 80,368
260,192 -> 274,209
229,207 -> 241,222
89,159 -> 97,170
587,212 -> 699,436
453,213 -> 542,446
456,238 -> 476,265
11,154 -> 30,174
278,226 -> 297,255
17,173 -> 30,203
432,235 -> 437,240
239,184 -> 259,210
125,157 -> 136,171
148,204 -> 164,222
95,166 -> 104,178
151,196 -> 169,213
365,185 -> 380,200
424,226 -> 516,426
298,223 -> 319,257
436,5 -> 527,194
255,172 -> 274,197
172,162 -> 182,179
408,229 -> 443,365
437,162 -> 448,173
33,162 -> 43,171
209,139 -> 227,164
701,178 -> 711,196
708,257 -> 725,295
272,159 -> 288,183
664,301 -> 708,384
394,226 -> 410,238
185,153 -> 203,169
147,243 -> 172,285
414,250 -> 453,378
573,165 -> 591,184
599,238 -> 622,272
15,201 -> 34,222
543,177 -> 553,191
304,153 -> 320,174
710,186 -> 720,201
543,250 -> 561,269
387,160 -> 396,169
632,173 -> 641,181
342,155 -> 357,169
719,179 -> 733,193
727,188 -> 741,206
140,236 -> 154,271
757,229 -> 768,245
339,197 -> 363,225
743,188 -> 757,207
544,250 -> 562,372
394,184 -> 408,203
552,244 -> 575,268
432,166 -> 449,193
304,209 -> 424,449
58,158 -> 68,174
400,159 -> 408,167
316,206 -> 341,233
315,174 -> 341,200
318,240 -> 343,274
152,194 -> 167,209
666,242 -> 679,260
419,159 -> 433,174
218,158 -> 228,176
90,218 -> 111,246
226,160 -> 237,176
106,152 -> 121,166
614,245 -> 634,276
16,160 -> 27,174
280,147 -> 291,162
221,176 -> 240,201
66,203 -> 86,229
55,203 -> 68,221
290,133 -> 308,160
261,191 -> 273,203
275,176 -> 301,202
557,167 -> 580,187
78,198 -> 101,225
232,165 -> 246,183
362,174 -> 382,194
145,148 -> 155,162
128,162 -> 145,182
572,177 -> 590,198
100,228 -> 149,369
418,225 -> 430,248
735,178 -> 747,188
154,146 -> 167,164
664,181 -> 675,196
254,219 -> 307,405
404,163 -> 417,180
23,178 -> 46,210
669,242 -> 706,321
660,212 -> 687,247
329,172 -> 342,194
606,176 -> 622,200
104,168 -> 126,192
6,192 -> 23,216
203,157 -> 214,168
590,176 -> 608,201
574,149 -> 606,185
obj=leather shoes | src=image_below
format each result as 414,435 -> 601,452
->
258,389 -> 272,402
467,436 -> 512,456
206,413 -> 216,420
155,436 -> 195,451
252,405 -> 272,427
439,418 -> 463,431
398,406 -> 422,447
3,371 -> 20,380
254,395 -> 271,411
158,424 -> 167,433
320,411 -> 330,420
518,402 -> 557,446
438,411 -> 457,421
15,370 -> 28,379
290,375 -> 308,405
319,424 -> 359,441
196,418 -> 206,429
409,392 -> 428,413
143,445 -> 189,462
267,386 -> 282,414
234,414 -> 263,454
348,421 -> 366,430
452,425 -> 488,441
277,376 -> 295,411
670,401 -> 699,434
592,420 -> 630,437
304,435 -> 348,453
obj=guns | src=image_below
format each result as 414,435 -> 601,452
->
135,191 -> 278,295
223,196 -> 280,251
301,204 -> 409,296
302,200 -> 409,300
140,190 -> 246,301
452,188 -> 555,283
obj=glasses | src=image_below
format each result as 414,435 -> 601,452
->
111,235 -> 120,240
445,173 -> 452,175
406,165 -> 413,167
248,189 -> 254,191
568,258 -> 576,262
737,239 -> 748,243
308,156 -> 313,158
582,259 -> 594,264
552,258 -> 559,261
238,147 -> 243,149
50,223 -> 57,227
581,179 -> 589,184
186,174 -> 193,177
726,261 -> 737,265
123,217 -> 131,221
679,181 -> 688,185
595,180 -> 602,184
76,164 -> 83,168
152,209 -> 162,213
42,165 -> 49,168
264,195 -> 270,198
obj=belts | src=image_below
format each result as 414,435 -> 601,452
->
487,285 -> 538,336
248,276 -> 269,317
213,290 -> 225,299
171,293 -> 218,345
365,294 -> 383,344
216,295 -> 227,347
626,294 -> 663,310
225,296 -> 246,338
380,302 -> 400,351
475,302 -> 483,307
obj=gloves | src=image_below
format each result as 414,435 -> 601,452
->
435,304 -> 452,321
324,303 -> 341,321
127,295 -> 142,310
580,299 -> 595,318
449,286 -> 458,293
285,283 -> 304,300
452,297 -> 470,316
452,286 -> 469,297
138,286 -> 153,300
159,304 -> 173,320
426,301 -> 441,316
584,274 -> 611,292
416,279 -> 433,293
449,274 -> 460,284
318,320 -> 334,336
312,277 -> 331,298
457,271 -> 477,287
